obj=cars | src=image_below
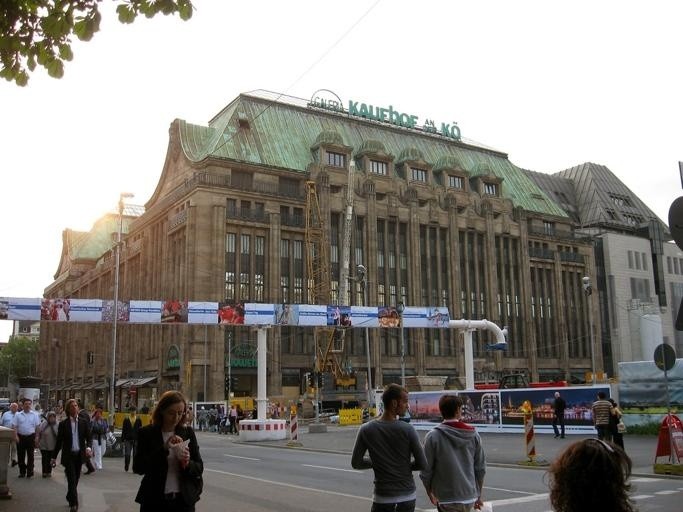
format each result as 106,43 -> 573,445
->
0,397 -> 11,409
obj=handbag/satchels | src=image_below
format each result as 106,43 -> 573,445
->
105,431 -> 116,447
178,424 -> 203,506
616,418 -> 626,434
469,502 -> 491,512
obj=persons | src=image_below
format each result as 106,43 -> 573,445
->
41,299 -> 70,322
0,390 -> 279,512
275,302 -> 294,326
161,300 -> 182,322
592,390 -> 615,442
542,437 -> 638,511
334,306 -> 348,324
420,395 -> 486,511
382,310 -> 400,328
397,405 -> 410,423
551,391 -> 566,438
363,407 -> 369,419
351,383 -> 428,511
608,398 -> 625,452
218,302 -> 245,325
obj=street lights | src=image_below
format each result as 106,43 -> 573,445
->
396,300 -> 407,391
109,192 -> 138,433
580,276 -> 598,386
357,263 -> 373,409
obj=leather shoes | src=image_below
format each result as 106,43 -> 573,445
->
70,504 -> 78,512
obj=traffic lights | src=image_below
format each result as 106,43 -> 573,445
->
231,376 -> 241,392
306,372 -> 314,387
317,372 -> 324,389
225,377 -> 229,392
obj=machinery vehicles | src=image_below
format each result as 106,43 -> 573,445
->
293,180 -> 359,419
496,372 -> 531,390
226,395 -> 275,421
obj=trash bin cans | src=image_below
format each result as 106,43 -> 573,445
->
0,426 -> 17,499
